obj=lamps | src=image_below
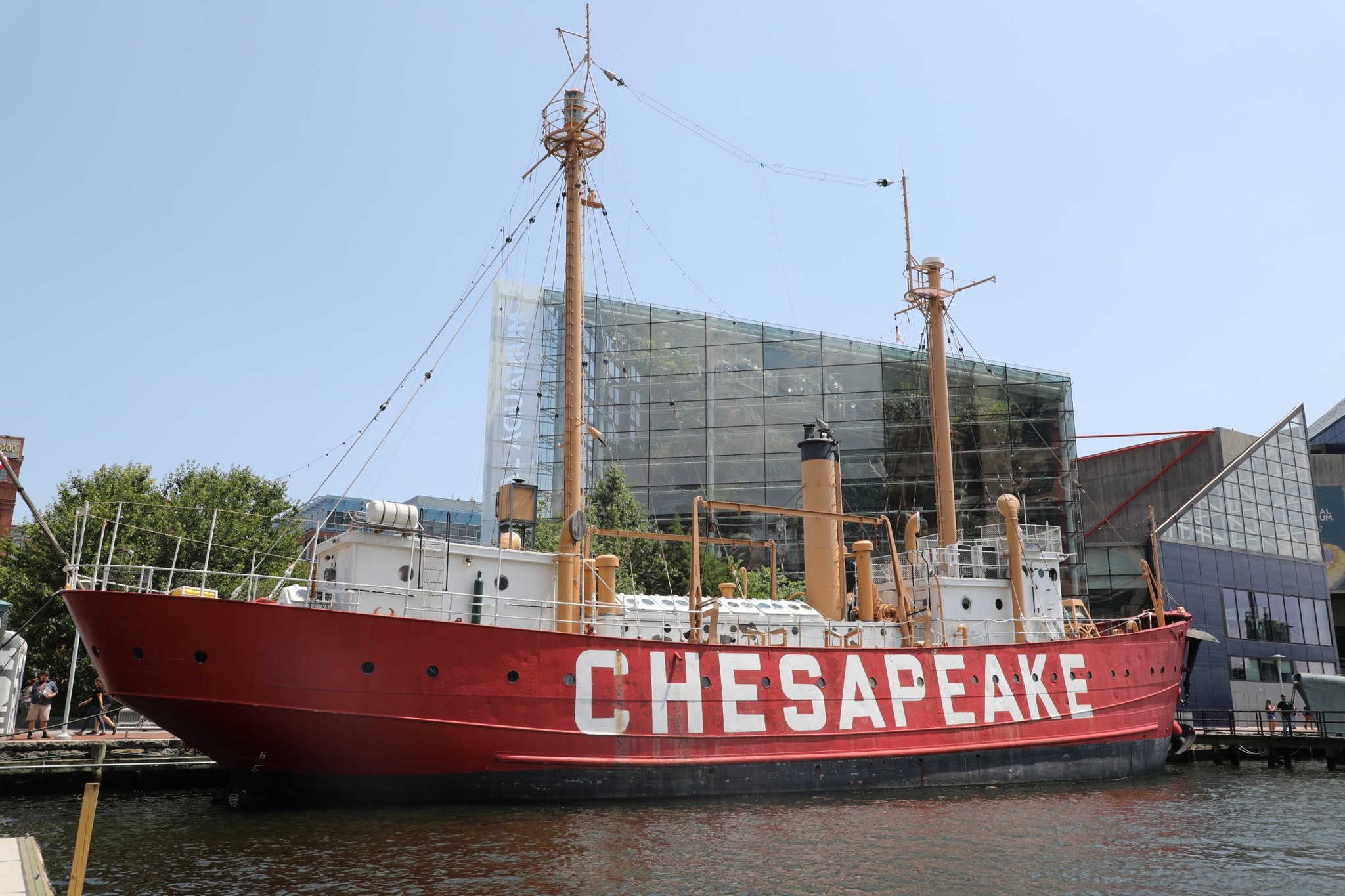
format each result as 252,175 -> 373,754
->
325,555 -> 334,561
465,557 -> 473,568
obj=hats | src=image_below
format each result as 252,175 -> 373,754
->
1281,694 -> 1286,697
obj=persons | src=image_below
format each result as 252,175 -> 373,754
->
1265,698 -> 1276,736
1302,703 -> 1315,730
1277,694 -> 1293,736
73,677 -> 117,736
20,669 -> 59,739
14,678 -> 39,733
1290,703 -> 1297,729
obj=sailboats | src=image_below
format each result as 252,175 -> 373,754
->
1,0 -> 1221,807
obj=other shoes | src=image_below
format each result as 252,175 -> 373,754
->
112,724 -> 118,735
1293,726 -> 1296,729
27,734 -> 32,740
42,733 -> 51,738
86,730 -> 99,735
98,731 -> 106,735
73,731 -> 82,736
1304,724 -> 1307,729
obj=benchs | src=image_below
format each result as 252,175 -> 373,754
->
112,707 -> 164,738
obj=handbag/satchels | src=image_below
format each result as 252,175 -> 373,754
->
1303,709 -> 1308,716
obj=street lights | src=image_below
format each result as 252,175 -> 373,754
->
1271,653 -> 1287,696
